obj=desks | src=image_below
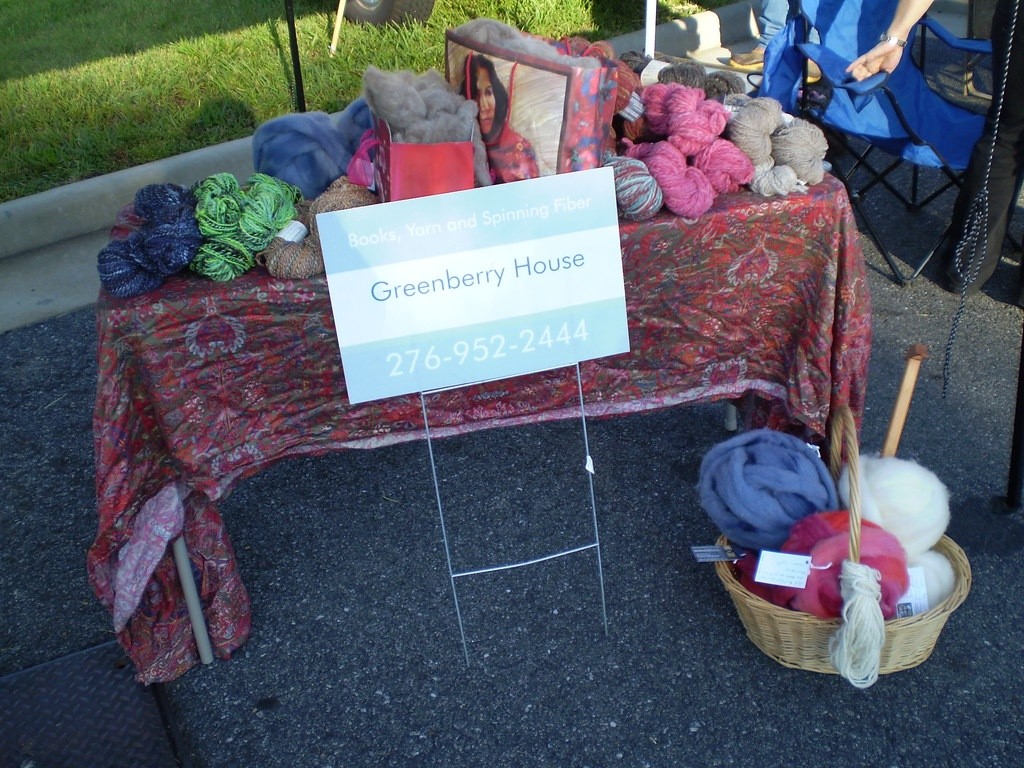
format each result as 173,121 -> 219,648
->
86,168 -> 872,766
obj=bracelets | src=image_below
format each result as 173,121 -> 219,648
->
879,32 -> 907,47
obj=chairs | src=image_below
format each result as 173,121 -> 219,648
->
797,0 -> 994,285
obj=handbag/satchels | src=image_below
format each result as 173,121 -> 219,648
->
444,22 -> 620,184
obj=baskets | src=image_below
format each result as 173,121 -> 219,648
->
714,405 -> 972,676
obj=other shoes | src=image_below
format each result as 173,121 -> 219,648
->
730,46 -> 766,73
808,60 -> 821,83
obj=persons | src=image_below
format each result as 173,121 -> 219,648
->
458,53 -> 541,184
730,0 -> 821,82
845,0 -> 1024,296
